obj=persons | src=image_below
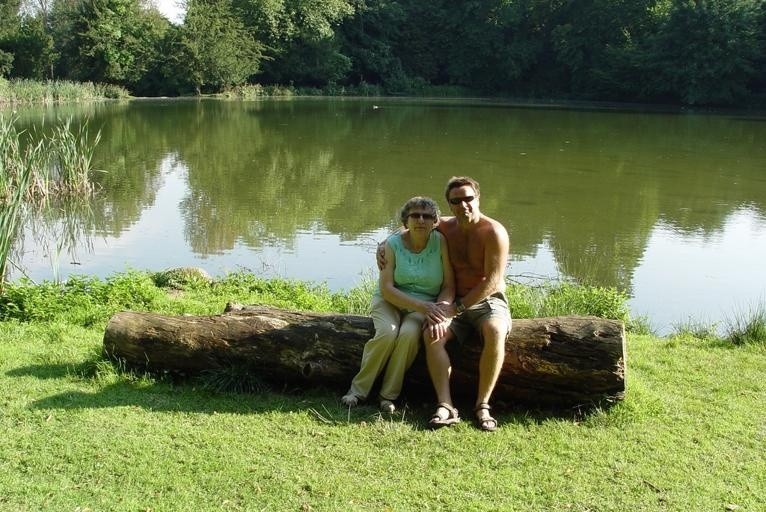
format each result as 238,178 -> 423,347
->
340,196 -> 457,415
376,175 -> 513,431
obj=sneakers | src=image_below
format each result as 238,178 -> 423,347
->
341,390 -> 360,409
376,394 -> 395,412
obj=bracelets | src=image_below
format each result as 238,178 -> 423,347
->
456,298 -> 467,314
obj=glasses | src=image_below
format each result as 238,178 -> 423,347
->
406,213 -> 434,220
447,196 -> 477,204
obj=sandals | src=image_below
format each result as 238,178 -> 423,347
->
472,403 -> 498,431
429,402 -> 460,427
